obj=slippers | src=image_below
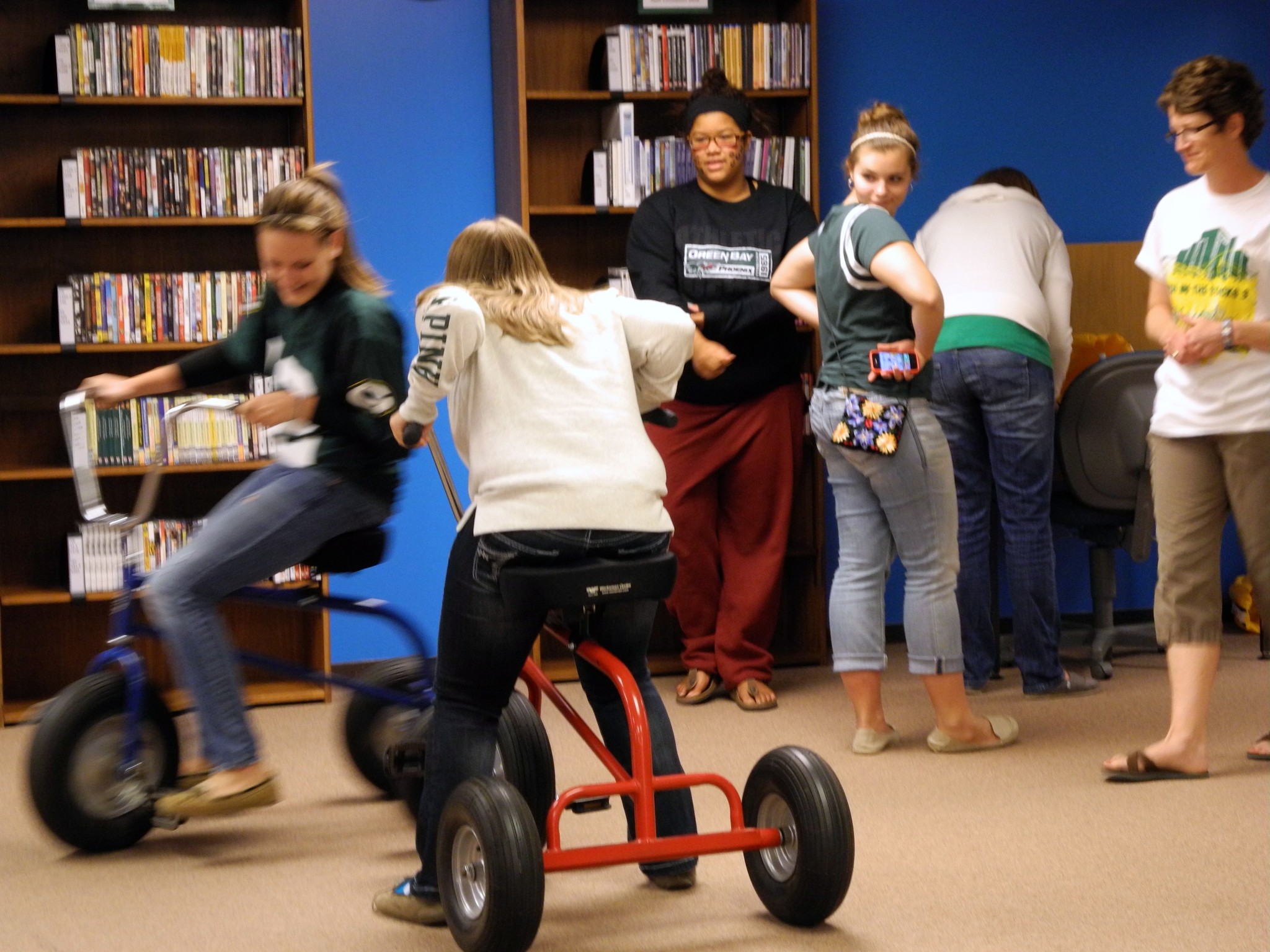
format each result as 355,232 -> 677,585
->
674,667 -> 725,704
1247,730 -> 1270,760
729,681 -> 777,711
1102,750 -> 1208,781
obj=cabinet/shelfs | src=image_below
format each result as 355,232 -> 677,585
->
0,0 -> 830,730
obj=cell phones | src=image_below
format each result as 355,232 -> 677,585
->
869,349 -> 919,377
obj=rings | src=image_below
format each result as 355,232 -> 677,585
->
1172,350 -> 1179,357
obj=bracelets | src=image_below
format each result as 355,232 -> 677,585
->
1222,319 -> 1233,349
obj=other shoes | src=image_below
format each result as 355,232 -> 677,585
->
375,884 -> 444,925
648,864 -> 694,889
1025,672 -> 1100,700
964,681 -> 982,695
851,723 -> 899,753
927,714 -> 1018,752
155,773 -> 280,820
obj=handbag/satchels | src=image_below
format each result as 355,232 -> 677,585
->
831,393 -> 908,455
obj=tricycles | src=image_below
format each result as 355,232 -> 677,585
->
393,409 -> 858,952
20,391 -> 439,855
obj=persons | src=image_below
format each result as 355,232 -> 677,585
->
1101,56 -> 1270,780
370,215 -> 702,929
771,103 -> 1020,751
76,164 -> 407,824
914,168 -> 1100,697
625,69 -> 819,709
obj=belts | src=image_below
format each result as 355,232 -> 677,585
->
816,378 -> 838,392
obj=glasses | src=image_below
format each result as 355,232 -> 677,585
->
686,130 -> 747,150
1166,116 -> 1220,147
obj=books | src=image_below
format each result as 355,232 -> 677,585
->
69,270 -> 264,344
80,518 -> 207,592
593,102 -> 810,212
605,18 -> 811,92
272,563 -> 322,585
65,22 -> 306,97
72,146 -> 305,220
86,375 -> 284,466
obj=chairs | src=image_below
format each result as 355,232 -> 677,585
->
985,348 -> 1238,683
